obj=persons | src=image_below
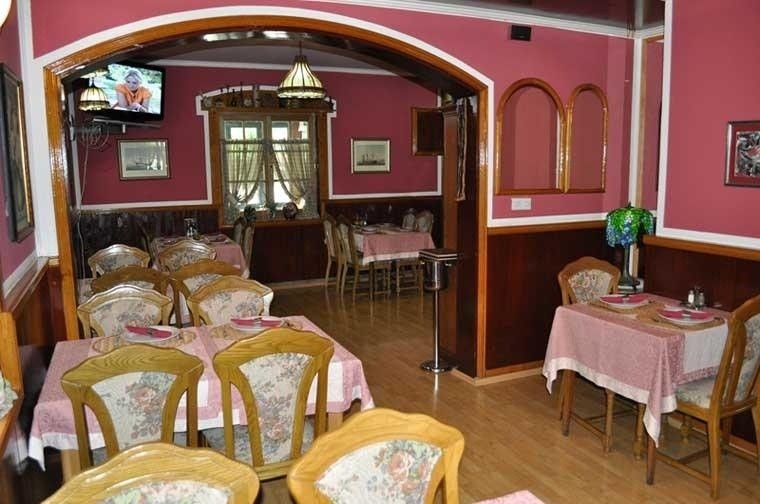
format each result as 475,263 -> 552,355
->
116,68 -> 152,114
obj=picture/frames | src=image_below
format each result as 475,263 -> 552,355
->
0,64 -> 35,245
117,138 -> 172,181
725,120 -> 760,187
412,106 -> 444,157
350,137 -> 392,175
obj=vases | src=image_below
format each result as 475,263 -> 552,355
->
618,244 -> 641,287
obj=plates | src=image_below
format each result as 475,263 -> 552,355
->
121,325 -> 180,342
228,315 -> 284,332
363,228 -> 377,231
599,294 -> 649,309
657,307 -> 714,326
156,233 -> 231,247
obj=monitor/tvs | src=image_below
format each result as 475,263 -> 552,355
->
78,59 -> 166,123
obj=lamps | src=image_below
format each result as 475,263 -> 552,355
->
77,75 -> 110,112
276,39 -> 329,101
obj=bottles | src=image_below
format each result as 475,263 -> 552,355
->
695,289 -> 705,311
688,288 -> 694,303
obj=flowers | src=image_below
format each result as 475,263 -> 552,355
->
606,207 -> 655,249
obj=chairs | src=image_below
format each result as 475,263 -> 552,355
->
321,208 -> 437,309
632,295 -> 760,500
39,440 -> 262,504
555,256 -> 637,452
287,406 -> 466,504
